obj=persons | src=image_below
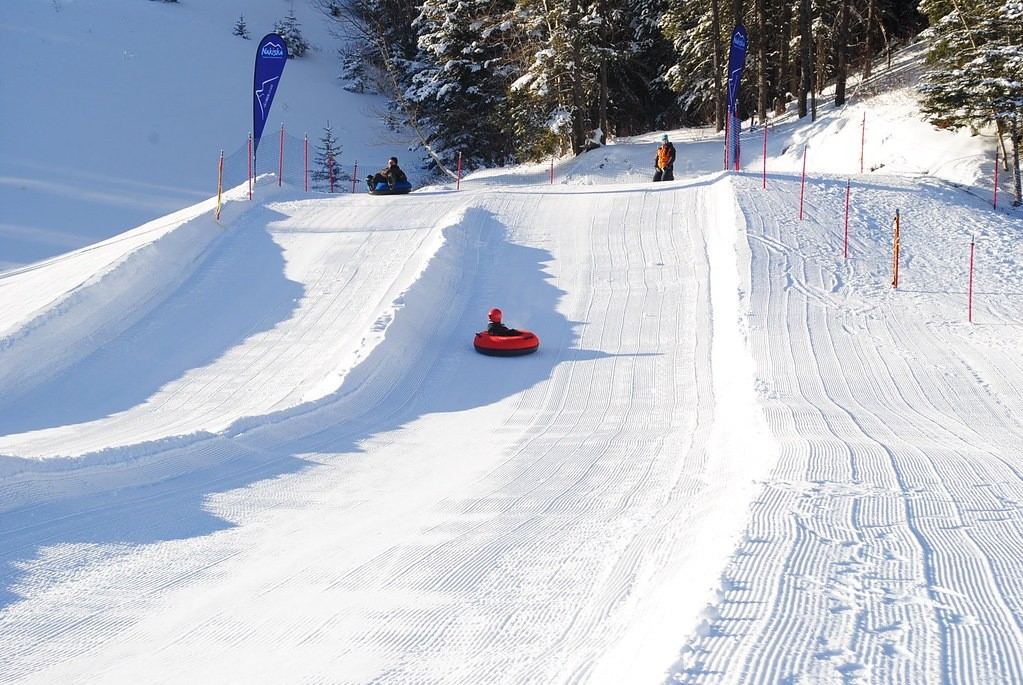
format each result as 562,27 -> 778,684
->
487,309 -> 518,335
366,157 -> 406,190
653,135 -> 676,181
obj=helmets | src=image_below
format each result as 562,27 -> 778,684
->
488,308 -> 503,322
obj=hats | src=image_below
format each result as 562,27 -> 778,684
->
662,134 -> 668,141
390,157 -> 398,165
